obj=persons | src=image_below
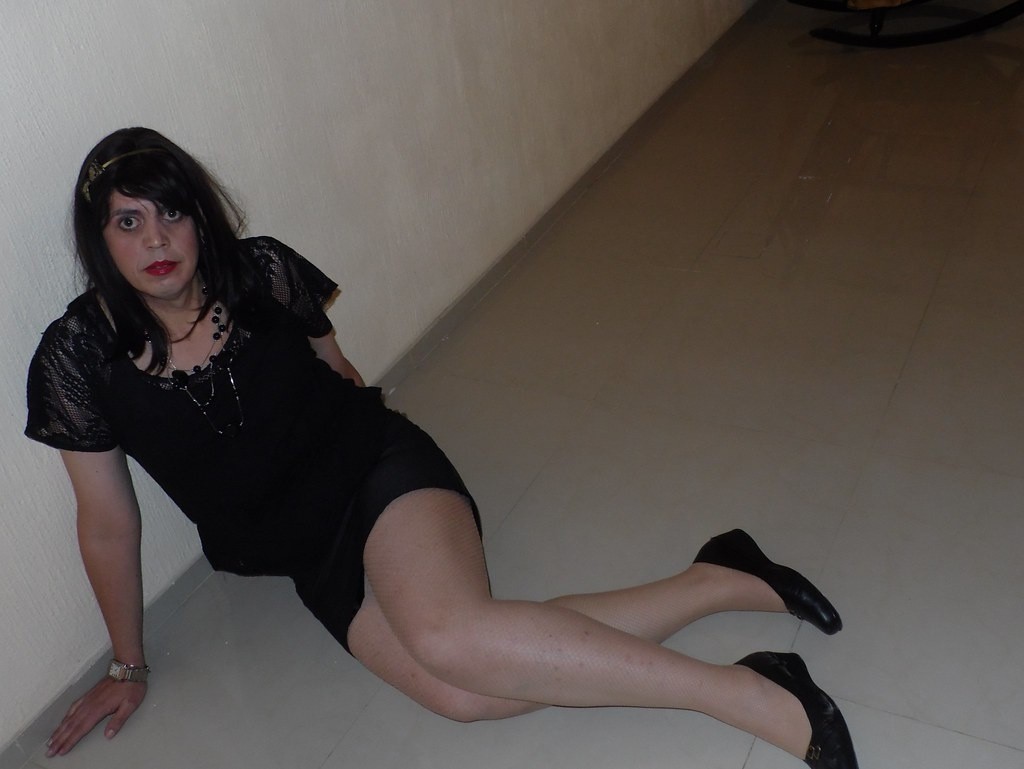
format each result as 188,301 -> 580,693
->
25,127 -> 862,769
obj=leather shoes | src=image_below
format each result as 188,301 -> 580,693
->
692,528 -> 843,635
734,650 -> 859,769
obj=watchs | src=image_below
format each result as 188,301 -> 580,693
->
107,659 -> 150,682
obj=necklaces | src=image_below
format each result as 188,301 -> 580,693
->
143,280 -> 245,437
